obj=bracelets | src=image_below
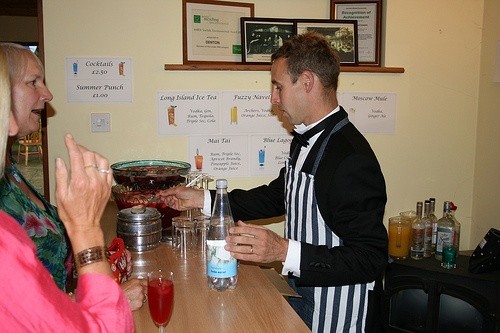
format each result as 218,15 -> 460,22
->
74,246 -> 108,268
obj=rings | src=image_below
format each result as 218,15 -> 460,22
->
86,164 -> 97,168
143,294 -> 148,302
251,246 -> 253,252
99,169 -> 109,174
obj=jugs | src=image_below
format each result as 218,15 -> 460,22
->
388,210 -> 418,261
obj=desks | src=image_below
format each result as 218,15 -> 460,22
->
100,202 -> 312,333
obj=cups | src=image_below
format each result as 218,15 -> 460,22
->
440,246 -> 458,269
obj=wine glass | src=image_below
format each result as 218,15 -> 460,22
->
171,171 -> 211,251
147,270 -> 176,333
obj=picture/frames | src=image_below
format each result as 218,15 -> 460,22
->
240,17 -> 294,63
294,19 -> 359,66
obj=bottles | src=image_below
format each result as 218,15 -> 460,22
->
207,179 -> 238,292
409,197 -> 461,262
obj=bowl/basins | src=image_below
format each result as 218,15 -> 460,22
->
111,186 -> 183,233
110,160 -> 192,190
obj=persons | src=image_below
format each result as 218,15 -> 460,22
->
156,33 -> 390,333
0,46 -> 134,333
0,42 -> 149,312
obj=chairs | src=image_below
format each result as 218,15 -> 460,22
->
18,119 -> 43,167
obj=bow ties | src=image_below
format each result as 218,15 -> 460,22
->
289,110 -> 340,148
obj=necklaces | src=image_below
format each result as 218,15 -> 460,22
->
4,160 -> 21,183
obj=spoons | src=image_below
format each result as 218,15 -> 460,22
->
130,195 -> 161,215
